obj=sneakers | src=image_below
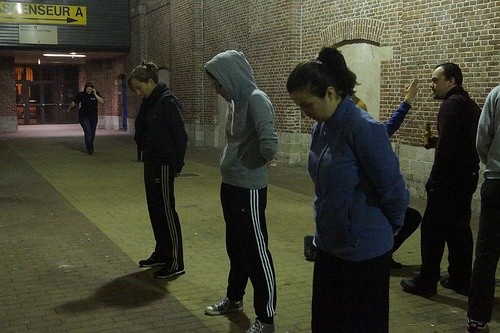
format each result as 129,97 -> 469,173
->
440,270 -> 469,290
465,320 -> 490,333
139,252 -> 167,266
304,235 -> 316,259
205,297 -> 243,315
400,278 -> 435,297
153,263 -> 185,279
391,259 -> 403,269
244,320 -> 274,333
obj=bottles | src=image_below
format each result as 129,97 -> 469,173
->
423,123 -> 432,149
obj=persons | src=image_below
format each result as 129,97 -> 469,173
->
304,79 -> 423,272
399,63 -> 482,297
465,87 -> 500,333
287,48 -> 410,333
66,83 -> 105,155
202,50 -> 278,333
126,61 -> 187,280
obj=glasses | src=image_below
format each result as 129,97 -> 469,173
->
86,83 -> 93,87
211,83 -> 222,89
